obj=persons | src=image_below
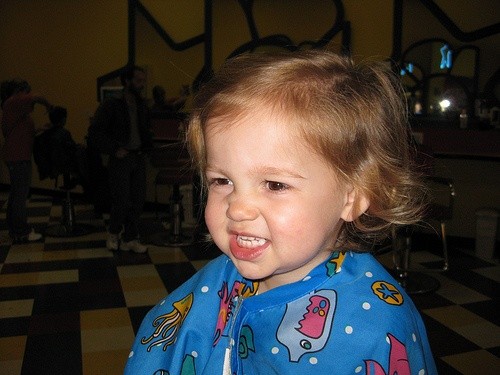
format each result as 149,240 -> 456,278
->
124,47 -> 439,375
0,63 -> 156,252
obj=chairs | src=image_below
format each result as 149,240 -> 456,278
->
32,137 -> 97,238
379,160 -> 452,294
149,117 -> 193,248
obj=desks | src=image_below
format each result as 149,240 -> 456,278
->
407,119 -> 500,159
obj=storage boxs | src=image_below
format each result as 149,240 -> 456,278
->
475,210 -> 498,261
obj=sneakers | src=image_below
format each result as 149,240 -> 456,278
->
120,239 -> 149,253
107,234 -> 120,250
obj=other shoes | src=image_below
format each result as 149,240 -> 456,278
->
13,228 -> 42,242
